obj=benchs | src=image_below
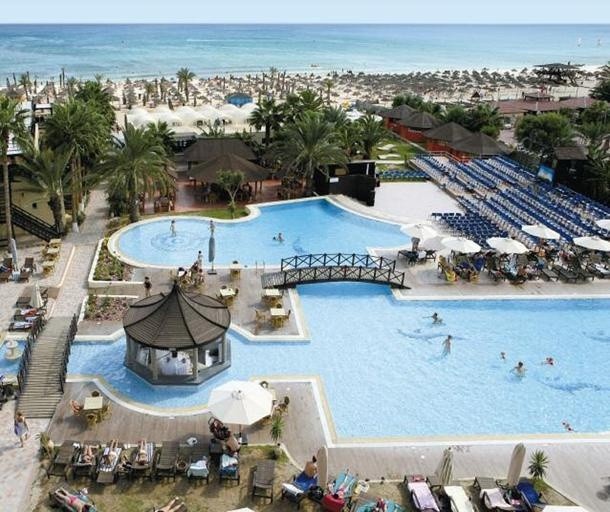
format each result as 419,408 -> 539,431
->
376,152 -> 610,283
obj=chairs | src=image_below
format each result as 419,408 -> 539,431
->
0,255 -> 36,282
46,439 -> 549,511
68,391 -> 112,431
9,287 -> 50,331
169,261 -> 292,336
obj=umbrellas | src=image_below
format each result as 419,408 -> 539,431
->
506,442 -> 527,497
431,445 -> 455,484
486,236 -> 528,254
441,236 -> 481,254
10,238 -> 20,273
29,281 -> 43,309
595,218 -> 610,232
521,222 -> 561,240
400,222 -> 441,242
573,235 -> 610,252
208,232 -> 216,263
206,379 -> 272,438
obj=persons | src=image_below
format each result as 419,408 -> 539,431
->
170,219 -> 177,236
448,239 -> 600,283
442,335 -> 452,357
198,250 -> 204,267
55,487 -> 91,511
81,442 -> 100,463
138,439 -> 148,465
14,411 -> 30,447
224,430 -> 240,453
103,438 -> 119,466
423,313 -> 438,324
302,455 -> 320,480
151,496 -> 185,512
143,276 -> 153,297
277,232 -> 285,242
180,262 -> 206,289
208,220 -> 216,234
508,362 -> 526,378
326,467 -> 359,499
